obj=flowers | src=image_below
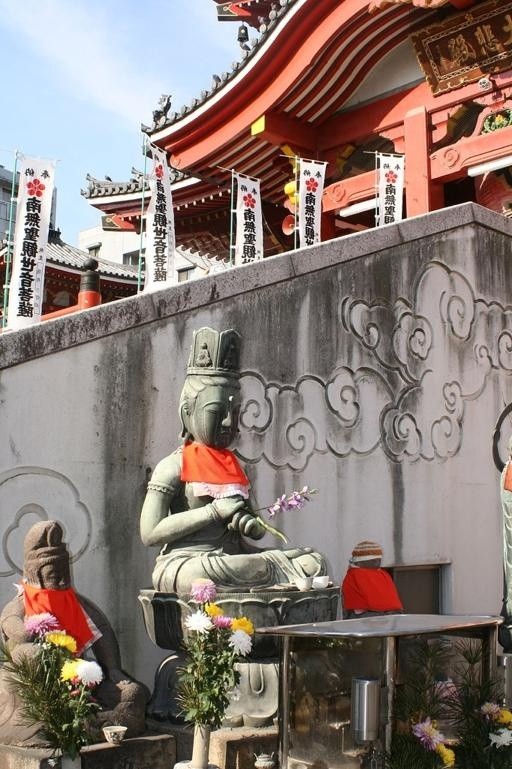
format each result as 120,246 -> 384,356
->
251,485 -> 318,520
400,635 -> 512,769
0,612 -> 102,767
173,576 -> 255,741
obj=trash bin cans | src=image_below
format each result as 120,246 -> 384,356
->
352,676 -> 381,740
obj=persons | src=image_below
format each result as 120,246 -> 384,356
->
0,517 -> 152,752
134,323 -> 323,596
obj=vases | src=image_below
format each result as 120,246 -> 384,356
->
174,722 -> 221,769
56,754 -> 84,769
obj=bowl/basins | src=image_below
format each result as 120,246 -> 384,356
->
294,577 -> 314,593
102,726 -> 128,744
312,576 -> 329,588
